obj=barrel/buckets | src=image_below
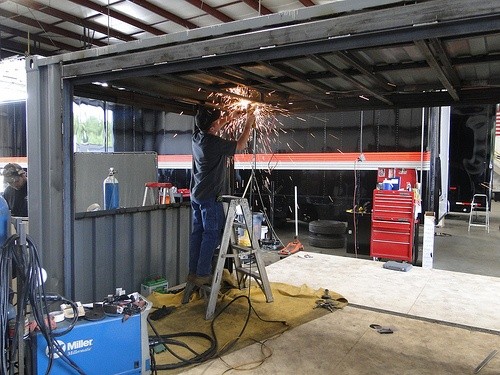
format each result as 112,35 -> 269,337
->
238,212 -> 262,241
261,226 -> 268,240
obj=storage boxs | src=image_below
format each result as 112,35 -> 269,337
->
370,189 -> 415,264
150,289 -> 196,308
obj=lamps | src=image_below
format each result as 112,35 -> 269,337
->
359,95 -> 369,101
176,107 -> 183,115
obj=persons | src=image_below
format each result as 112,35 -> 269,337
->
186,103 -> 255,284
3,163 -> 29,217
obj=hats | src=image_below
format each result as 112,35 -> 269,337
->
3,162 -> 23,183
195,107 -> 220,133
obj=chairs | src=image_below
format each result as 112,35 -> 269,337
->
467,194 -> 490,234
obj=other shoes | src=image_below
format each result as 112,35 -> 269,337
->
195,275 -> 224,285
187,273 -> 197,282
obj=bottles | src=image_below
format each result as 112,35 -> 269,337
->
406,182 -> 411,189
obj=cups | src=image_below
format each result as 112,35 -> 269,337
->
383,180 -> 393,190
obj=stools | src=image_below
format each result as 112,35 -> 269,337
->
142,183 -> 176,206
